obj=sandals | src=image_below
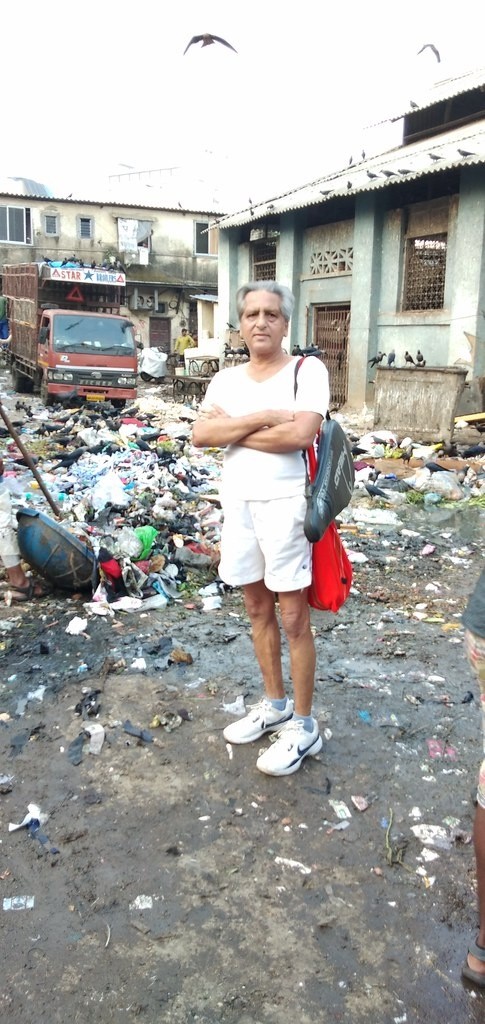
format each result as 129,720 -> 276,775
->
462,936 -> 485,986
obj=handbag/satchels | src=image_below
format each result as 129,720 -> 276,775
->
307,469 -> 352,613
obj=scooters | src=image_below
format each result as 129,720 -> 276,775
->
141,347 -> 167,384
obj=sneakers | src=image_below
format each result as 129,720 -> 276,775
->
223,697 -> 293,744
257,718 -> 323,777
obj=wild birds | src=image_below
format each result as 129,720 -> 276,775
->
348,431 -> 484,500
368,347 -> 426,369
247,194 -> 274,217
318,147 -> 478,197
0,382 -> 191,476
222,341 -> 250,359
416,42 -> 442,65
66,192 -> 74,199
225,322 -> 237,331
182,30 -> 238,56
41,253 -> 126,273
409,100 -> 419,110
291,343 -> 329,358
178,201 -> 183,209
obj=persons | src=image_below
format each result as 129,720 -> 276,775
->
461,568 -> 485,986
191,280 -> 330,777
173,328 -> 196,361
0,291 -> 9,349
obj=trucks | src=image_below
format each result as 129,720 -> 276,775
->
2,262 -> 143,408
